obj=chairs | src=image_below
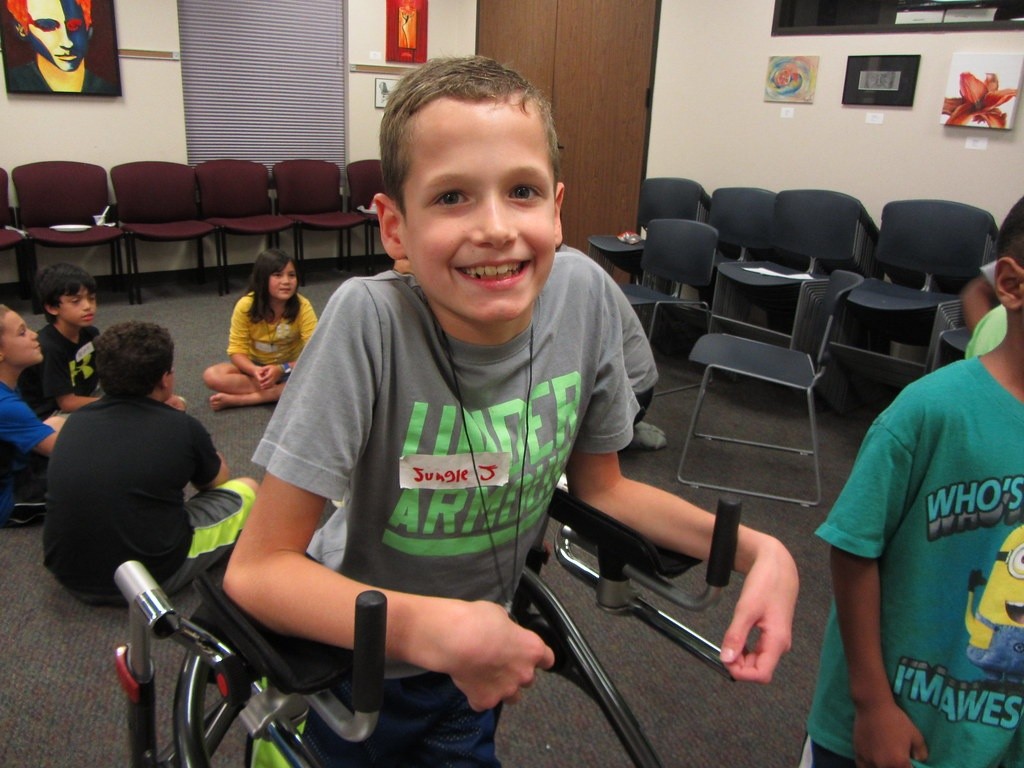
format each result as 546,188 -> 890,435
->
582,176 -> 1001,510
0,153 -> 397,316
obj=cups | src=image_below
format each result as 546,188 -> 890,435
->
94,215 -> 105,226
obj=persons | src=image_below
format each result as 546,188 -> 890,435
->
0,304 -> 69,528
202,247 -> 319,412
40,320 -> 262,609
18,261 -> 189,414
219,54 -> 803,768
797,193 -> 1024,768
959,259 -> 1001,340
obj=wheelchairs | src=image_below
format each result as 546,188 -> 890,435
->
112,462 -> 753,768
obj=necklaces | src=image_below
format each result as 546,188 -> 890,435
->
426,299 -> 536,615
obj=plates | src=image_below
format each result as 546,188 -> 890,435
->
357,205 -> 377,214
50,225 -> 93,231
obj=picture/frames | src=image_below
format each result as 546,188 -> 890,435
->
374,78 -> 402,109
841,54 -> 921,108
0,0 -> 122,97
